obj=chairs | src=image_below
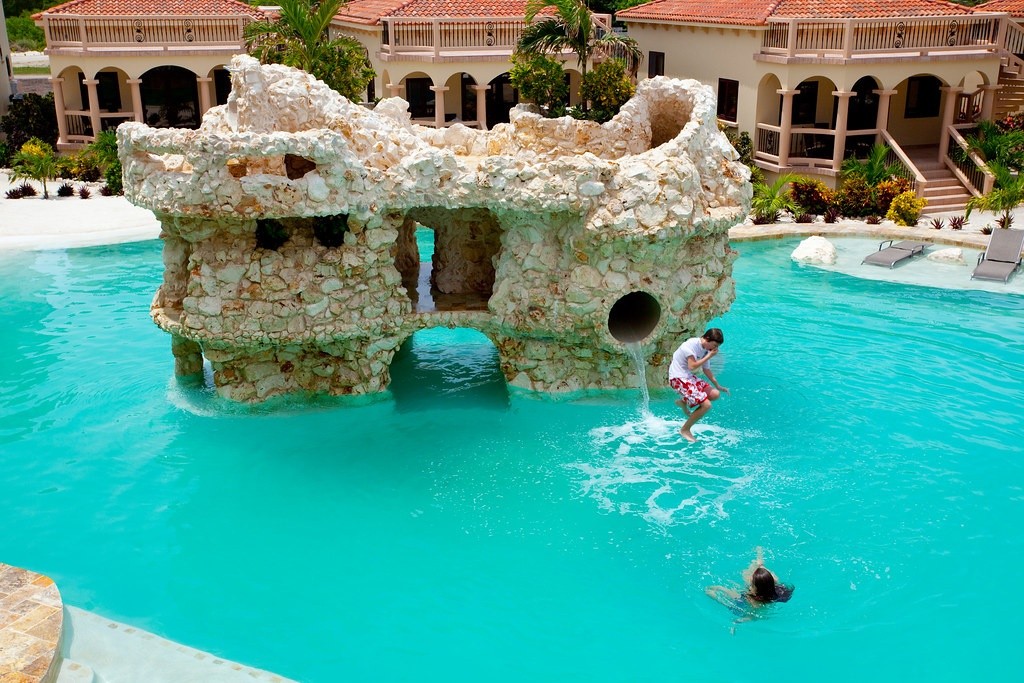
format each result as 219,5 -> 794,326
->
969,227 -> 1023,284
803,122 -> 830,156
861,239 -> 934,270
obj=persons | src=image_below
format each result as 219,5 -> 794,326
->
669,328 -> 730,442
749,567 -> 795,605
